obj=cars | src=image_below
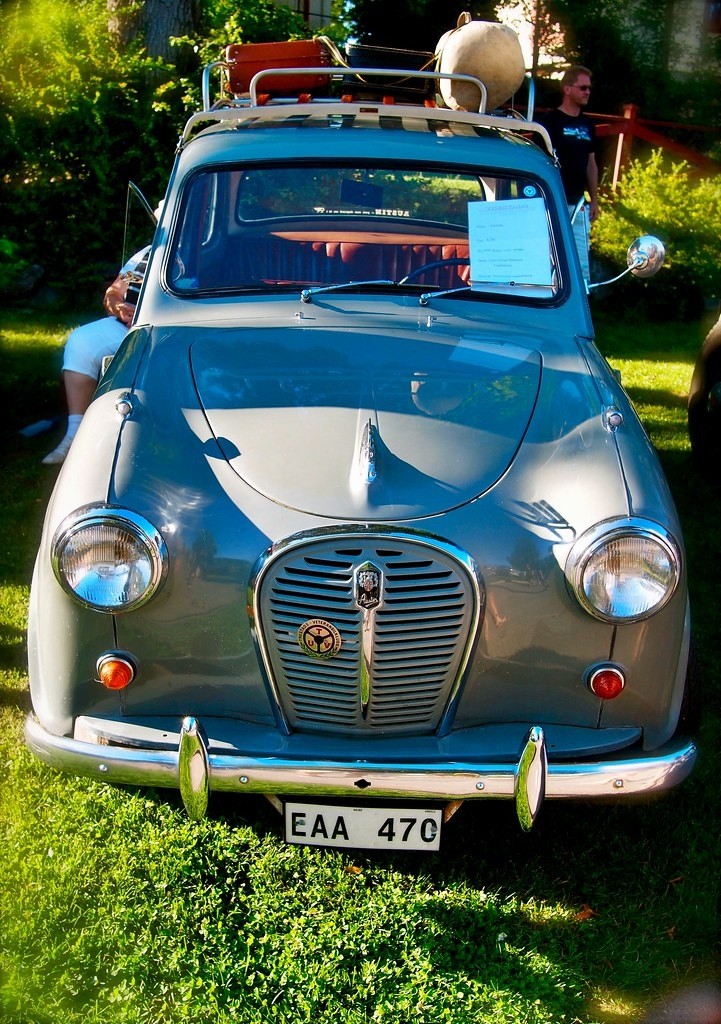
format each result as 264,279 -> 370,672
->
22,60 -> 700,853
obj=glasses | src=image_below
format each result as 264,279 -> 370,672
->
572,85 -> 591,91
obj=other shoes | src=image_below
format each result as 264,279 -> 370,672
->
43,450 -> 66,464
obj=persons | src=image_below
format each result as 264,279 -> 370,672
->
40,197 -> 164,464
533,65 -> 599,299
687,314 -> 721,494
